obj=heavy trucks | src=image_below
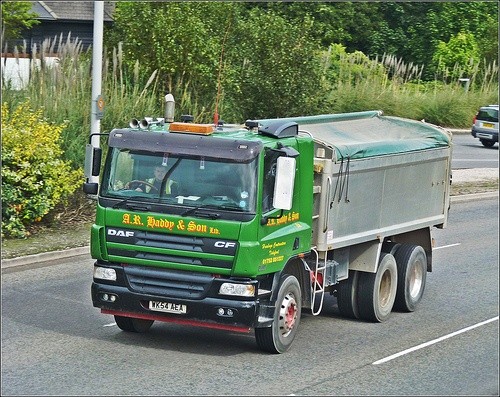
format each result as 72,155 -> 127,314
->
81,93 -> 453,351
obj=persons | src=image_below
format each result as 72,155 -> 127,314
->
134,162 -> 179,198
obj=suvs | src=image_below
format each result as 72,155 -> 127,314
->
470,104 -> 500,148
